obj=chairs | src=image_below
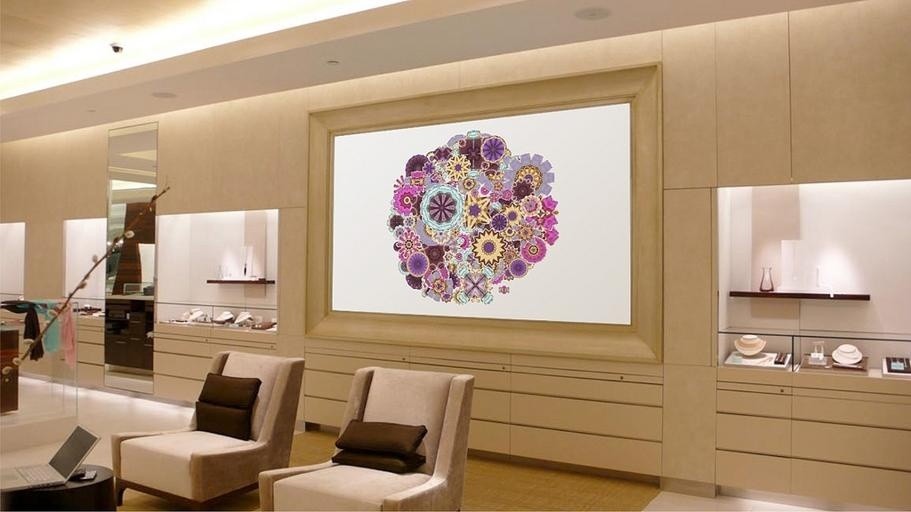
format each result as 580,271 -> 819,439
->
255,363 -> 474,512
111,351 -> 308,512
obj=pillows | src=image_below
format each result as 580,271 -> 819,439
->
195,400 -> 253,441
331,448 -> 427,474
335,417 -> 428,456
198,371 -> 262,407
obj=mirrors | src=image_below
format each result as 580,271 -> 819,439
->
105,121 -> 158,394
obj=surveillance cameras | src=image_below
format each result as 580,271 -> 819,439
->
111,42 -> 123,54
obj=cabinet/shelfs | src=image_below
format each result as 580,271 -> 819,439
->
710,179 -> 911,509
302,336 -> 660,481
59,209 -> 279,407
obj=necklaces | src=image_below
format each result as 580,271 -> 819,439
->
835,348 -> 861,361
734,337 -> 765,352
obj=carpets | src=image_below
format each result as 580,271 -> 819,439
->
0,373 -> 195,505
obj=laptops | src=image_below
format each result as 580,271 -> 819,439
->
0,424 -> 102,493
